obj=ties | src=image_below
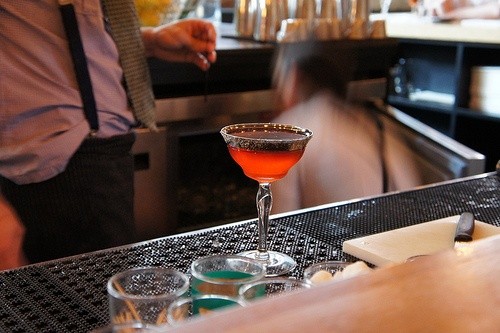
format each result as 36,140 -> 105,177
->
103,0 -> 160,134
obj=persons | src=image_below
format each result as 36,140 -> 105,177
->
262,54 -> 425,218
0,0 -> 219,267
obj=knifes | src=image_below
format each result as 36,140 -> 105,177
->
454,212 -> 474,246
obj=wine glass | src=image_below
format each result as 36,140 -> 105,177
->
222,123 -> 313,278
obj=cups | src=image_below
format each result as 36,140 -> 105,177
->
239,277 -> 312,333
191,255 -> 267,333
168,295 -> 244,333
106,267 -> 190,333
304,261 -> 365,333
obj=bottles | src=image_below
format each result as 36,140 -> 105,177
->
296,0 -> 317,41
342,0 -> 368,40
261,0 -> 288,42
321,0 -> 338,40
233,0 -> 258,37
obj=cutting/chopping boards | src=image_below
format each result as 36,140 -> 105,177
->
343,215 -> 500,333
